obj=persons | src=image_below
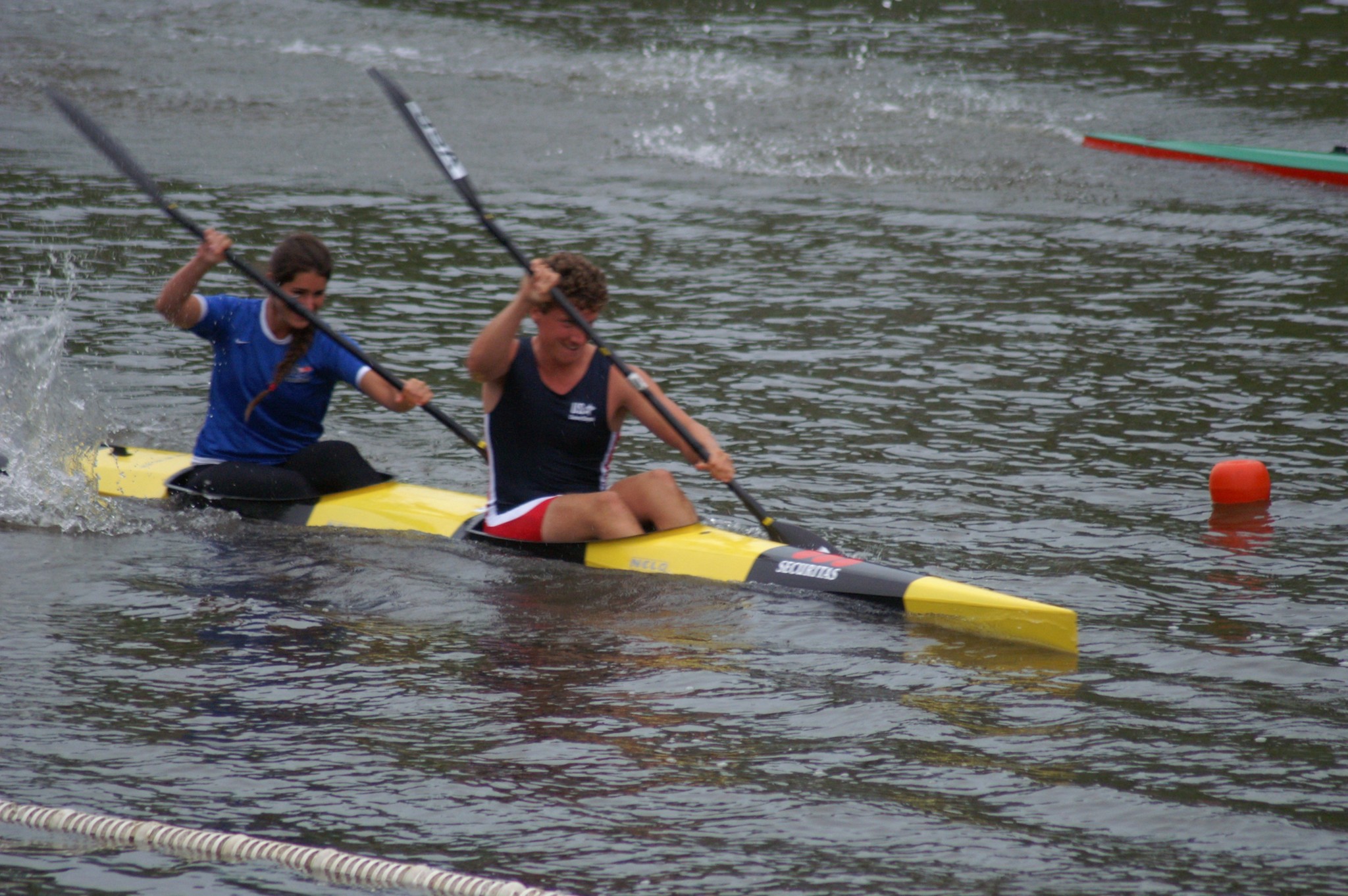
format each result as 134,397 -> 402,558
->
467,252 -> 735,540
155,225 -> 433,498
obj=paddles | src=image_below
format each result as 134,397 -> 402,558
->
48,89 -> 491,460
367,60 -> 842,559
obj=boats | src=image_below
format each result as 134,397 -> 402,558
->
1080,131 -> 1348,192
1,442 -> 1085,657
